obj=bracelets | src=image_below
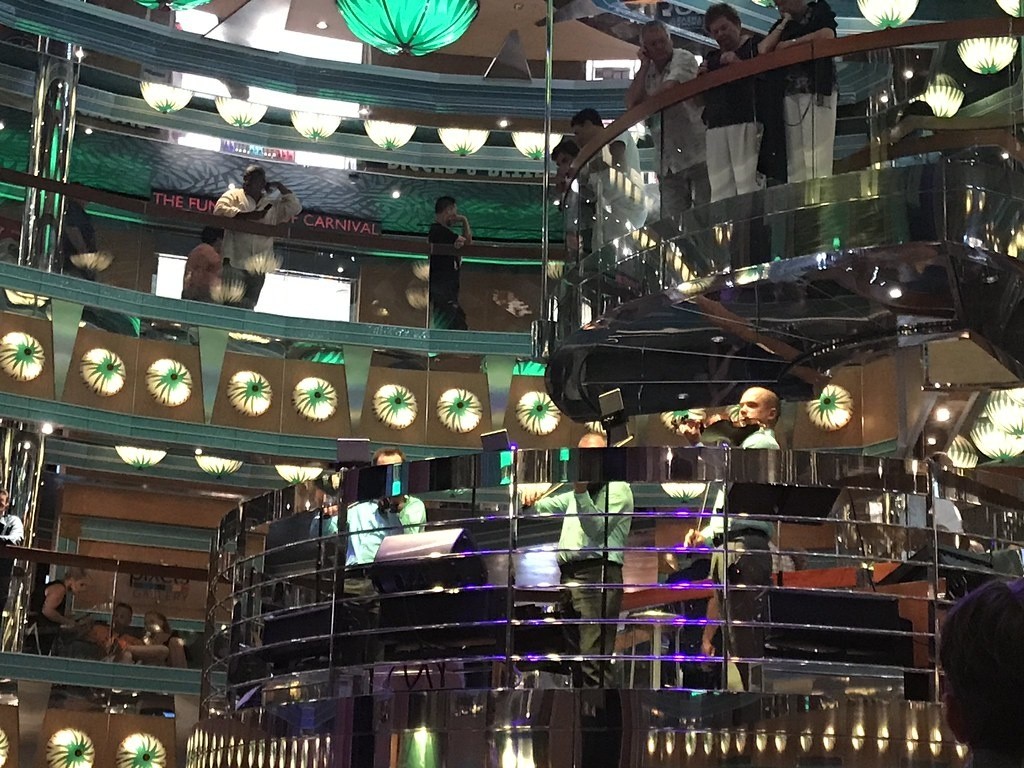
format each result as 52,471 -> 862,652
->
777,23 -> 785,30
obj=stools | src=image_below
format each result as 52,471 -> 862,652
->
631,610 -> 681,690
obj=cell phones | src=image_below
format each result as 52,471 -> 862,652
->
264,204 -> 272,210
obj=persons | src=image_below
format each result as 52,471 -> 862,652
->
182,224 -> 220,303
697,0 -> 841,201
215,166 -> 303,309
677,386 -> 791,692
937,576 -> 1024,768
27,564 -> 188,666
59,180 -> 102,278
521,433 -> 633,688
548,104 -> 647,312
628,16 -> 711,217
309,445 -> 429,614
424,192 -> 472,332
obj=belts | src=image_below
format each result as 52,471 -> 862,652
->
707,115 -> 756,127
559,559 -> 616,572
713,528 -> 766,546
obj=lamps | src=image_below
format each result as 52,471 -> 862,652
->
139,80 -> 192,113
1,331 -> 1024,500
439,128 -> 489,157
855,0 -> 921,28
335,0 -> 480,57
512,133 -> 560,159
957,37 -> 1017,77
215,96 -> 268,128
69,249 -> 112,273
363,119 -> 407,149
408,260 -> 435,312
995,0 -> 1024,20
909,73 -> 964,118
289,112 -> 341,139
116,732 -> 166,768
46,727 -> 96,768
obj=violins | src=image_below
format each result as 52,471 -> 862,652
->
375,496 -> 406,513
586,482 -> 604,490
672,412 -> 761,447
